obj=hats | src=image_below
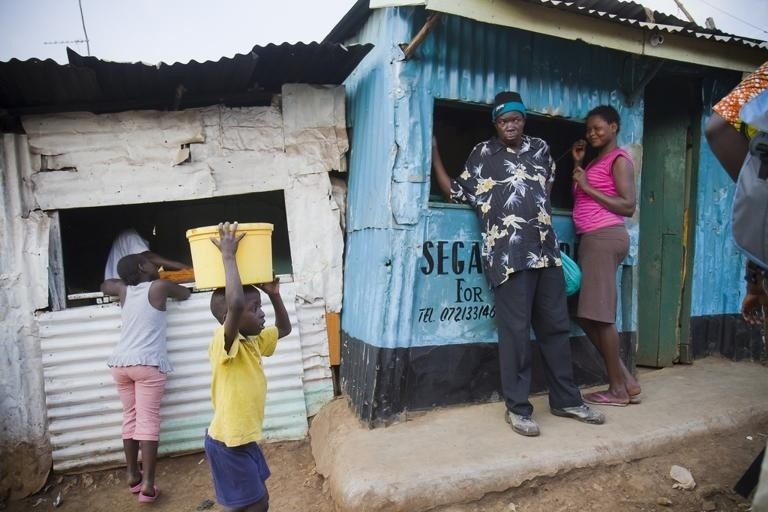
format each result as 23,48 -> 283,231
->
491,91 -> 526,122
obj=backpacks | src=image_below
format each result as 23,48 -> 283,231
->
729,87 -> 767,270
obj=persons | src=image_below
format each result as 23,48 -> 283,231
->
102,210 -> 192,281
428,90 -> 606,439
201,218 -> 294,512
98,252 -> 191,504
567,100 -> 645,410
702,42 -> 767,338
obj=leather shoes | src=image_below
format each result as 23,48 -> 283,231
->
550,403 -> 604,425
504,409 -> 539,437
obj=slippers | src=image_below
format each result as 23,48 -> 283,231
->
581,392 -> 628,407
129,470 -> 145,494
629,394 -> 642,404
139,485 -> 160,504
